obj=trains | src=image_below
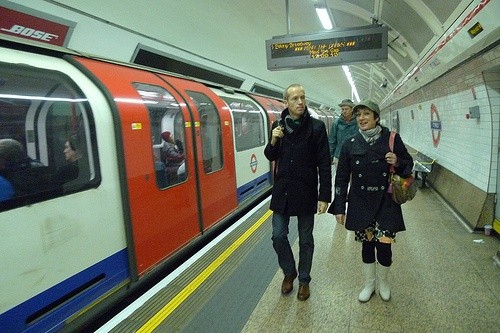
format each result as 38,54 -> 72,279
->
0,34 -> 341,333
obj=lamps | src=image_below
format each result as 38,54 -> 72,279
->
314,4 -> 333,30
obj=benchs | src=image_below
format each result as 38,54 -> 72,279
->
410,151 -> 437,188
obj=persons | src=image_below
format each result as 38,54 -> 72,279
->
264,84 -> 333,301
161,132 -> 186,182
328,99 -> 359,201
56,135 -> 91,186
327,98 -> 414,303
0,139 -> 58,197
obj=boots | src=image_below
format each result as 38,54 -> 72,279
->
358,258 -> 376,301
376,259 -> 394,301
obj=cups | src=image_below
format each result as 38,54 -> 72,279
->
484,225 -> 492,235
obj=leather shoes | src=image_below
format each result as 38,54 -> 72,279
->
281,270 -> 297,293
297,282 -> 310,300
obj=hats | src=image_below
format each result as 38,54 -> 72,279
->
338,98 -> 354,107
353,98 -> 380,116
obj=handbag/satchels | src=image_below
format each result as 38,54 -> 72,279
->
388,172 -> 417,204
